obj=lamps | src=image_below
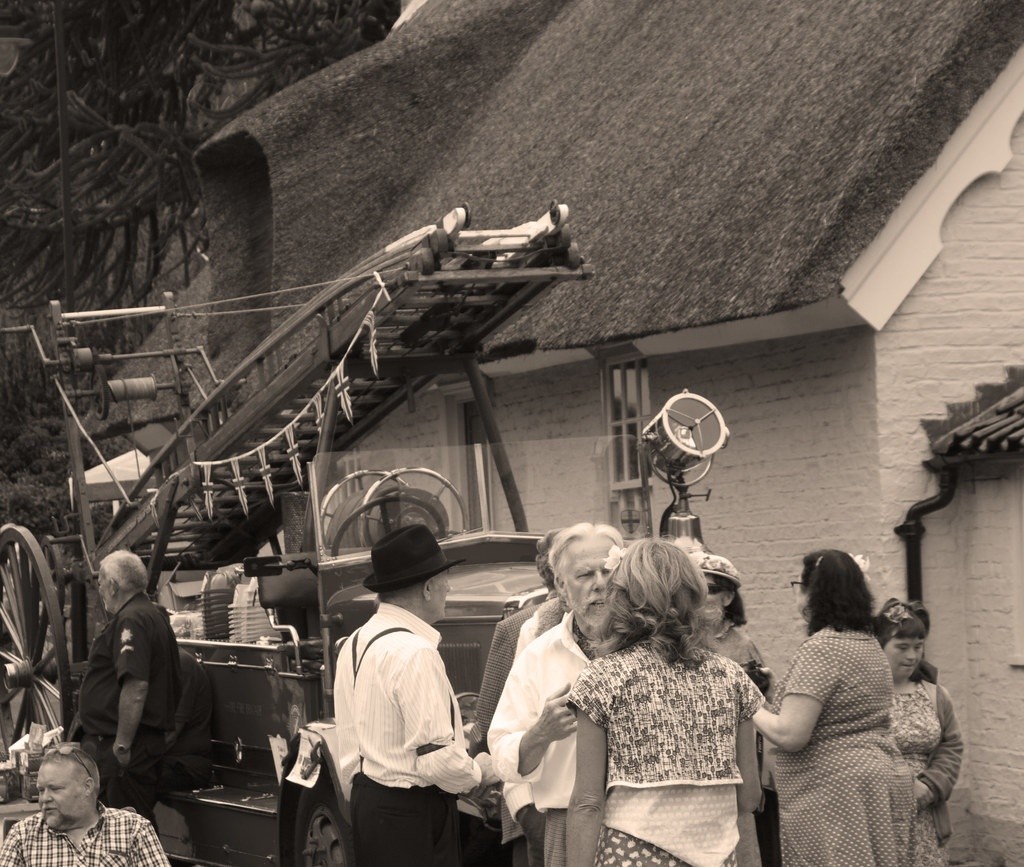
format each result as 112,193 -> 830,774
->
640,387 -> 729,538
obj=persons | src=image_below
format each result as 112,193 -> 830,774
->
79,550 -> 178,841
333,524 -> 501,867
476,522 -> 624,867
0,747 -> 172,867
753,550 -> 917,867
695,555 -> 781,867
566,540 -> 763,867
876,598 -> 963,867
154,603 -> 212,805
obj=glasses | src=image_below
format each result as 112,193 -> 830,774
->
43,744 -> 92,777
790,580 -> 806,588
706,582 -> 731,596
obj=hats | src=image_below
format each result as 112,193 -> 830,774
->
359,523 -> 471,593
689,550 -> 743,590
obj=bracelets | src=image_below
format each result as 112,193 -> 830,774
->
114,742 -> 130,752
